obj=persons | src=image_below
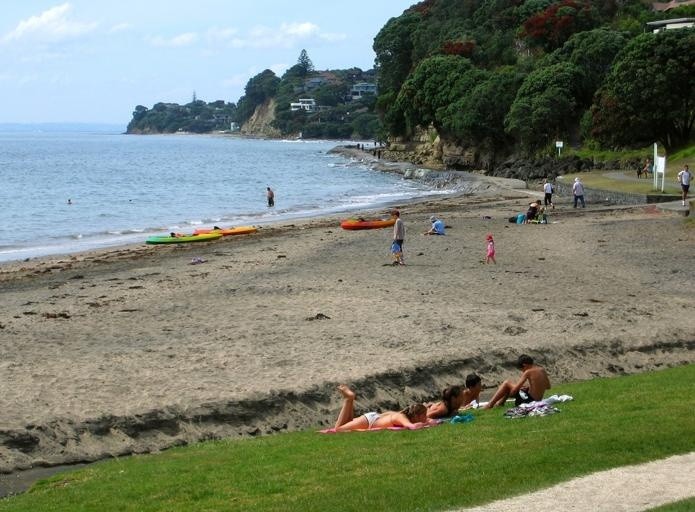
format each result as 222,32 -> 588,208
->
334,354 -> 551,430
169,231 -> 186,238
266,186 -> 275,206
390,209 -> 409,263
424,216 -> 446,236
572,177 -> 585,208
525,179 -> 556,221
677,164 -> 694,205
482,234 -> 498,265
641,157 -> 654,179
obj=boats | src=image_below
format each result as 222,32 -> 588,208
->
145,224 -> 259,244
338,213 -> 401,230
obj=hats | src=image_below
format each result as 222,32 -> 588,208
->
430,216 -> 437,222
575,177 -> 581,182
